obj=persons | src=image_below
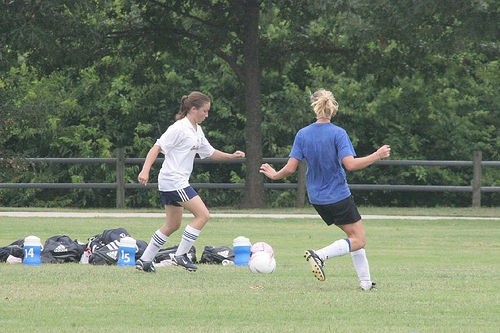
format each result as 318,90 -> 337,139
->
136,91 -> 246,272
259,89 -> 391,290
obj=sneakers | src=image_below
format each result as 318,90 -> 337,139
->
135,258 -> 156,272
171,253 -> 198,272
304,249 -> 325,281
362,282 -> 376,292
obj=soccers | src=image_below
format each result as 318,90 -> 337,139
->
250,242 -> 274,257
248,251 -> 276,274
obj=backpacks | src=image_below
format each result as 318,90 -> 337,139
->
201,246 -> 234,263
0,238 -> 24,261
156,244 -> 196,263
88,228 -> 149,265
43,234 -> 82,265
